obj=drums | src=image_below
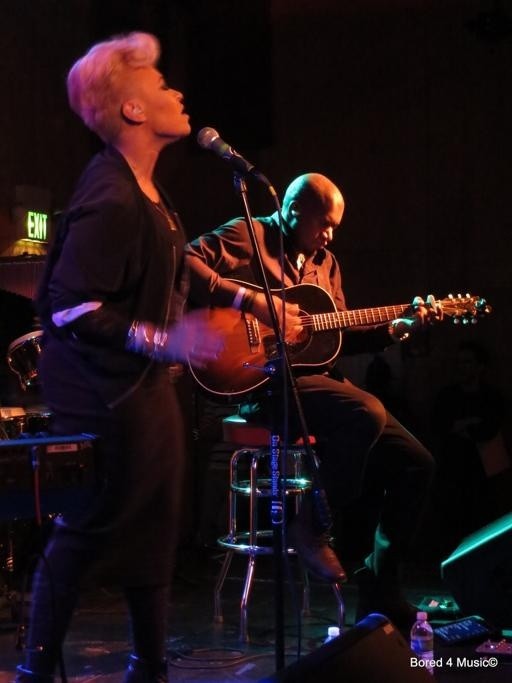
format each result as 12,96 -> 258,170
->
9,324 -> 42,388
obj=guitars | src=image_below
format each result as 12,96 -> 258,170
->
181,277 -> 489,394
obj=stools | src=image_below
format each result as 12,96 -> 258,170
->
213,415 -> 350,642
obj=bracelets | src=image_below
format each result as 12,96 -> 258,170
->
125,319 -> 169,365
231,282 -> 258,312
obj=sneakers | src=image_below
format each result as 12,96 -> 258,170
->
13,665 -> 54,683
125,654 -> 168,683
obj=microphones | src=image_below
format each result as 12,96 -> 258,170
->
197,127 -> 272,188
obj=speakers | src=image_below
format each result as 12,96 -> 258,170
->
268,614 -> 438,683
441,512 -> 512,628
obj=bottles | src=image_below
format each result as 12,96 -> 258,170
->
412,612 -> 435,676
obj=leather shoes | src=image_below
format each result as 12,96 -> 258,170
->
356,578 -> 431,629
296,522 -> 348,583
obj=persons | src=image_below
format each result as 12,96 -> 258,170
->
185,170 -> 462,624
11,31 -> 225,683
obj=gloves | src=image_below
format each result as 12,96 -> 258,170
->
124,308 -> 224,368
